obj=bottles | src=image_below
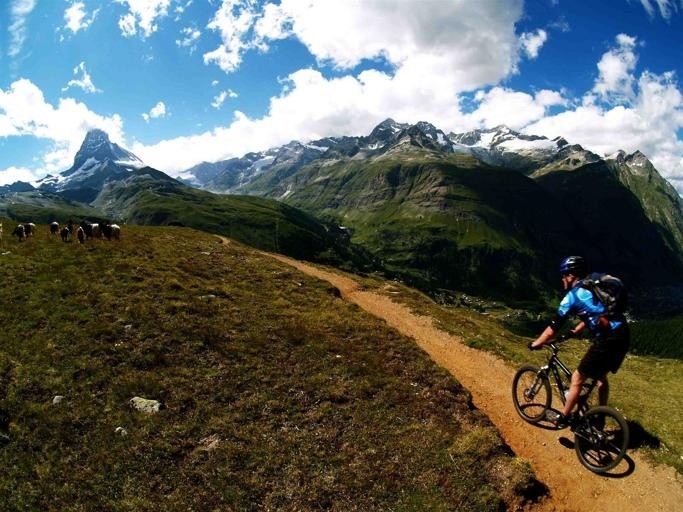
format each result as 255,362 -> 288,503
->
563,385 -> 570,399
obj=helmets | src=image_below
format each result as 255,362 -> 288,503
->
560,256 -> 584,275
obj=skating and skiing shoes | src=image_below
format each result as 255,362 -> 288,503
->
545,408 -> 568,429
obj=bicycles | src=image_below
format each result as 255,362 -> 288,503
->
512,333 -> 629,473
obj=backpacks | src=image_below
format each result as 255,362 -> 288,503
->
575,272 -> 629,318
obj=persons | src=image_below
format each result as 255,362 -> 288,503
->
530,255 -> 631,429
